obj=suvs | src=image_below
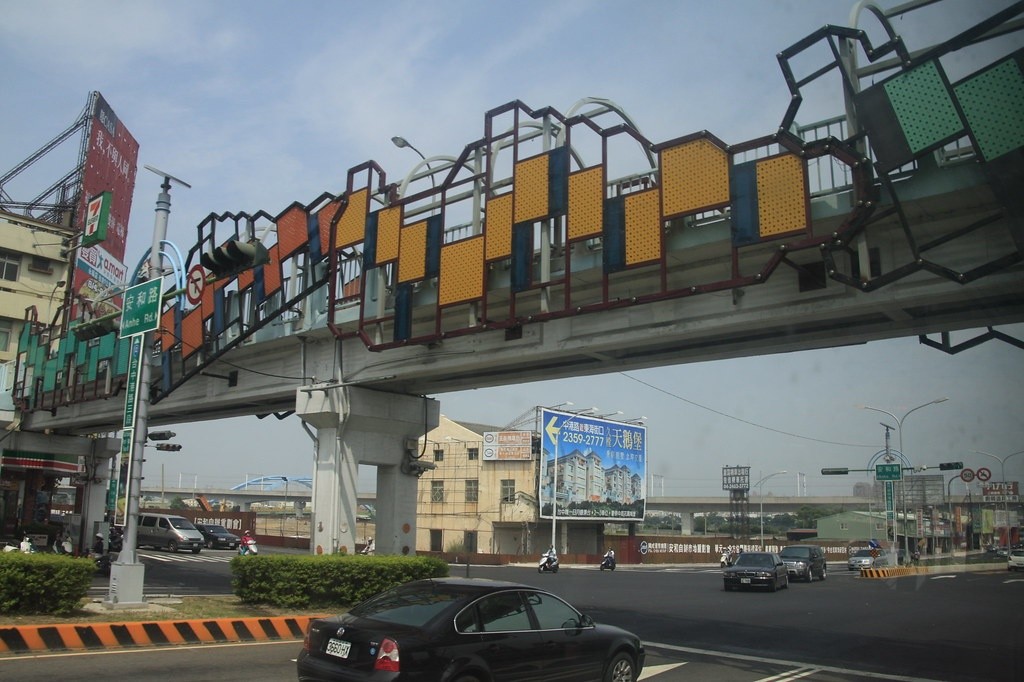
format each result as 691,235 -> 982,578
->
779,545 -> 827,583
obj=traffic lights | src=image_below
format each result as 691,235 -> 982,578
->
157,443 -> 182,452
939,462 -> 962,471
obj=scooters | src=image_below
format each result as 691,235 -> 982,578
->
537,553 -> 560,573
77,544 -> 113,575
600,556 -> 616,572
108,531 -> 124,551
52,530 -> 74,555
235,540 -> 259,557
1,529 -> 37,554
357,547 -> 370,555
719,554 -> 733,568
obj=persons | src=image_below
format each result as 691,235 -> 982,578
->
545,544 -> 556,568
914,548 -> 920,566
723,547 -> 744,562
366,537 -> 375,556
88,533 -> 104,560
241,530 -> 254,556
603,547 -> 615,567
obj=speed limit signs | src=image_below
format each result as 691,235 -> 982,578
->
960,468 -> 975,481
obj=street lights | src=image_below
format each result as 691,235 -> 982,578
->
758,471 -> 786,553
553,410 -> 596,551
281,477 -> 288,515
108,165 -> 192,611
392,136 -> 436,215
969,449 -> 1024,556
865,397 -> 950,560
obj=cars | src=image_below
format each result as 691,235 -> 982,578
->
723,553 -> 788,592
194,524 -> 242,549
847,548 -> 889,570
886,550 -> 918,565
988,543 -> 1024,571
297,575 -> 647,682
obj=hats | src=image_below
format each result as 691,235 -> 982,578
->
368,537 -> 373,540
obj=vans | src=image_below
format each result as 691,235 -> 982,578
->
137,512 -> 206,554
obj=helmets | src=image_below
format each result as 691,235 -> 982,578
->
96,533 -> 105,540
244,530 -> 251,533
